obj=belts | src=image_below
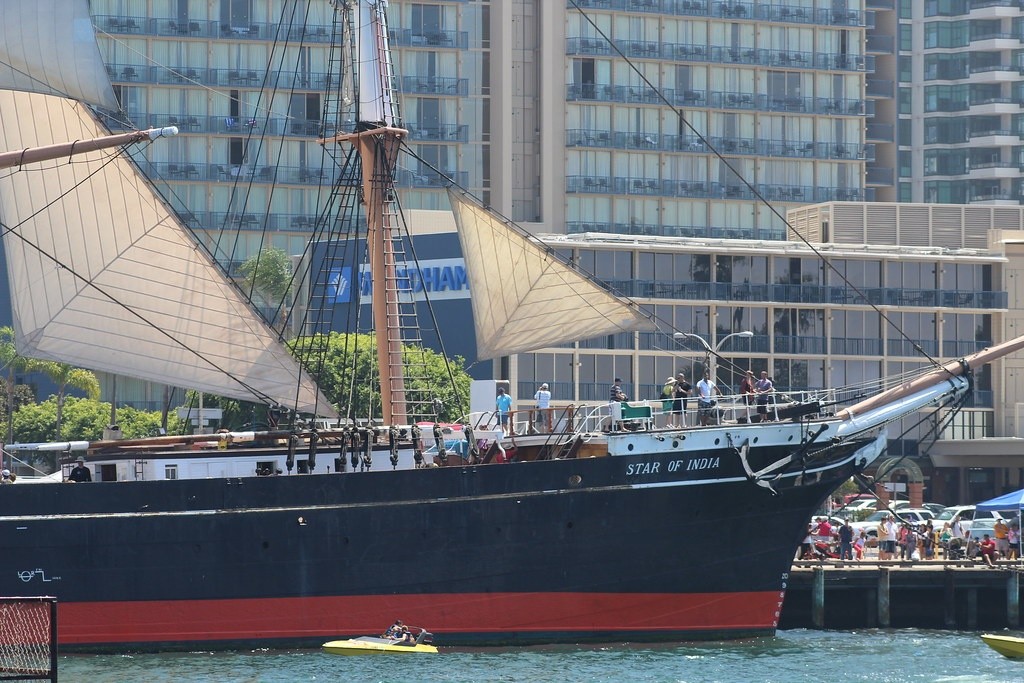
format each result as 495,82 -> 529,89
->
996,537 -> 1006,538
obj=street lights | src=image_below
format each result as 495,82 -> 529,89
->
674,330 -> 753,404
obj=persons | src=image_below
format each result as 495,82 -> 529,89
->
65,456 -> 92,483
976,534 -> 995,569
918,521 -> 935,560
886,514 -> 898,560
661,377 -> 677,429
533,383 -> 551,433
877,518 -> 889,560
894,524 -> 908,559
672,372 -> 693,428
494,387 -> 511,436
381,619 -> 411,643
853,531 -> 866,562
906,514 -> 917,522
794,517 -> 855,568
906,528 -> 916,560
1006,523 -> 1019,568
603,378 -> 629,433
0,469 -> 16,484
739,371 -> 758,419
994,519 -> 1011,569
949,515 -> 966,548
939,522 -> 953,560
696,371 -> 723,425
754,371 -> 772,423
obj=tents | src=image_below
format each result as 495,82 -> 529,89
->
964,489 -> 1024,568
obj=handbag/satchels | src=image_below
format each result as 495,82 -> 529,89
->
659,393 -> 668,402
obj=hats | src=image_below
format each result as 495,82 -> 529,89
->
542,383 -> 548,388
747,371 -> 754,374
2,470 -> 11,476
75,456 -> 86,461
615,378 -> 623,382
664,377 -> 678,384
996,517 -> 1003,521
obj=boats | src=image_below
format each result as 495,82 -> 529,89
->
979,634 -> 1024,662
321,625 -> 439,655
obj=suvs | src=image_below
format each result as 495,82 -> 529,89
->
810,499 -> 1018,548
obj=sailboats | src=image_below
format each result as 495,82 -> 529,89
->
1,1 -> 1023,650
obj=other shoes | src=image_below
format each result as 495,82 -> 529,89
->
988,565 -> 996,569
621,429 -> 631,432
604,429 -> 611,433
